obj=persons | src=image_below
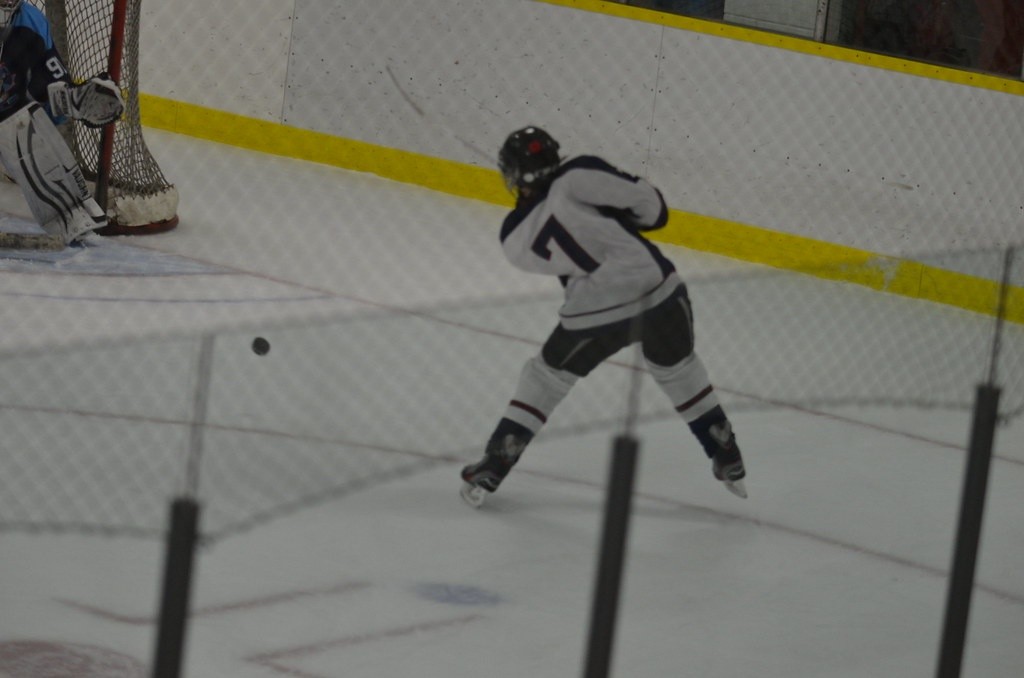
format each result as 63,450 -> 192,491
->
458,126 -> 748,507
0,0 -> 126,243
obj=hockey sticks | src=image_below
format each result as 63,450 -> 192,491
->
384,61 -> 506,170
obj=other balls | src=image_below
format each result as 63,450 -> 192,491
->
251,336 -> 272,356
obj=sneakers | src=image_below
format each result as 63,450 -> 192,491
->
706,417 -> 747,499
461,435 -> 527,506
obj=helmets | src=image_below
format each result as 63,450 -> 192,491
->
498,125 -> 560,199
0,0 -> 23,28
